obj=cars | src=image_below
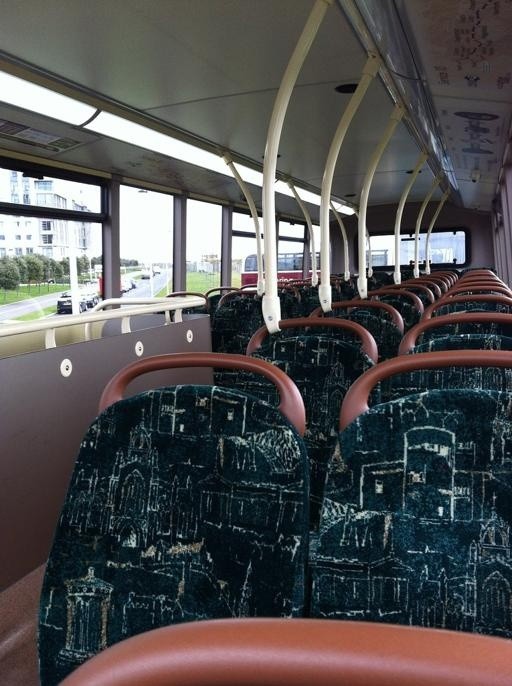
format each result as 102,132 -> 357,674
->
56,275 -> 137,312
141,265 -> 160,279
48,274 -> 69,284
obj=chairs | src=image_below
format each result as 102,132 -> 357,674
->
36,266 -> 511,683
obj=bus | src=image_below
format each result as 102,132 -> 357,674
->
241,251 -> 323,289
